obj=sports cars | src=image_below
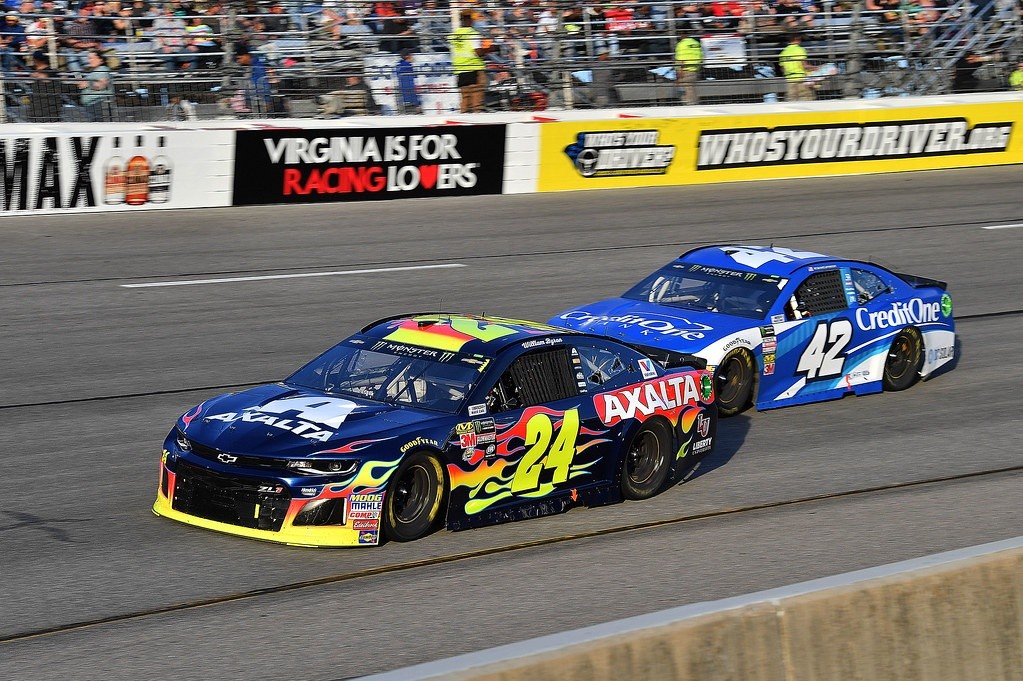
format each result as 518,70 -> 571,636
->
153,311 -> 716,548
546,244 -> 955,416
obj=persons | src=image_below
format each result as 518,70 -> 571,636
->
1,0 -> 1023,123
